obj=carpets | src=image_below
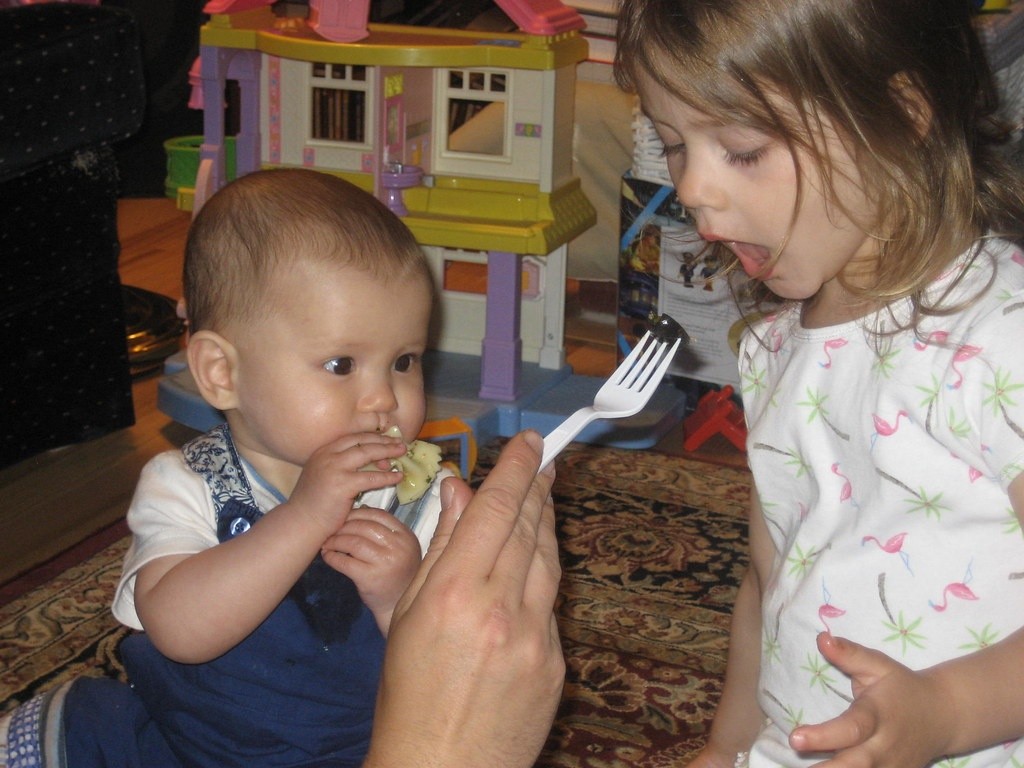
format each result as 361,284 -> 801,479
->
0,435 -> 754,768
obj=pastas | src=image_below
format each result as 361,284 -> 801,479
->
358,425 -> 442,505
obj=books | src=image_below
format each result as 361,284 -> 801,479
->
562,0 -> 619,64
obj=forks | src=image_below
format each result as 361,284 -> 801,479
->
537,329 -> 682,478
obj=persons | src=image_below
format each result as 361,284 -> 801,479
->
0,169 -> 456,768
614,0 -> 1023,768
360,429 -> 566,768
